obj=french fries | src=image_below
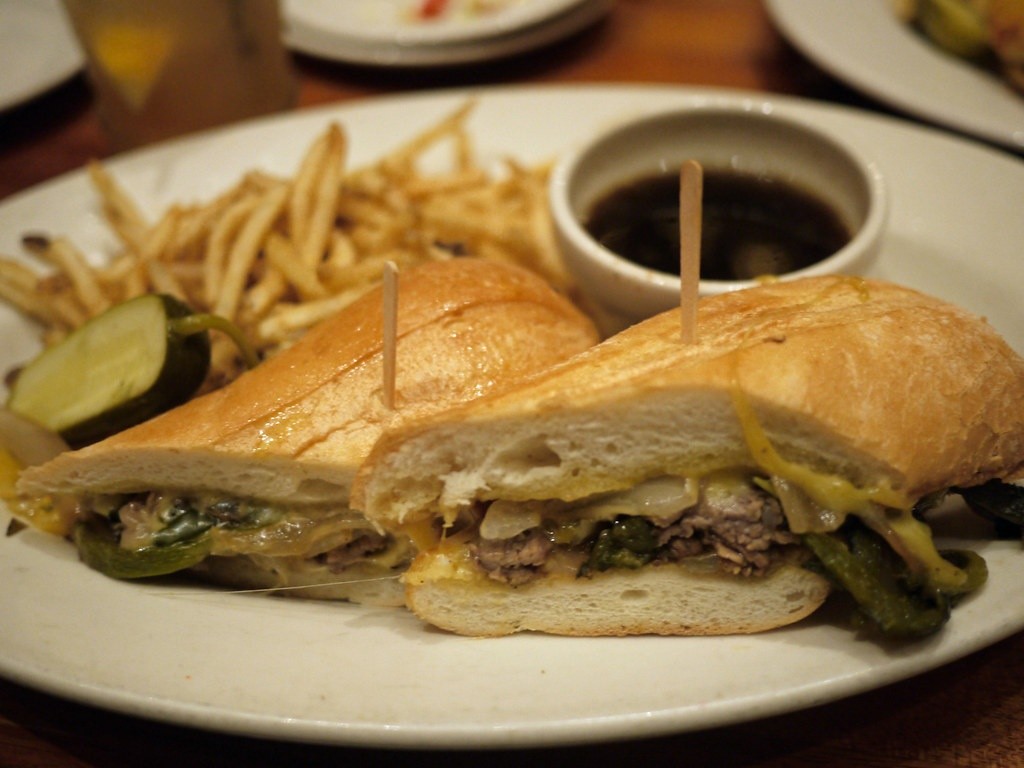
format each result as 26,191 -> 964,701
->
0,98 -> 576,410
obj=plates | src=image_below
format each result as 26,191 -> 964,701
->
0,80 -> 1024,746
768,0 -> 1024,146
278,0 -> 612,67
0,0 -> 88,112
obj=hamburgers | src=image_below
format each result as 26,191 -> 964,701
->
13,256 -> 609,608
341,270 -> 1024,641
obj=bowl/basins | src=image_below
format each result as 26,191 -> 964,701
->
549,103 -> 888,341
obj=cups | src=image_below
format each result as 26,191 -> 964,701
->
60,0 -> 301,153
